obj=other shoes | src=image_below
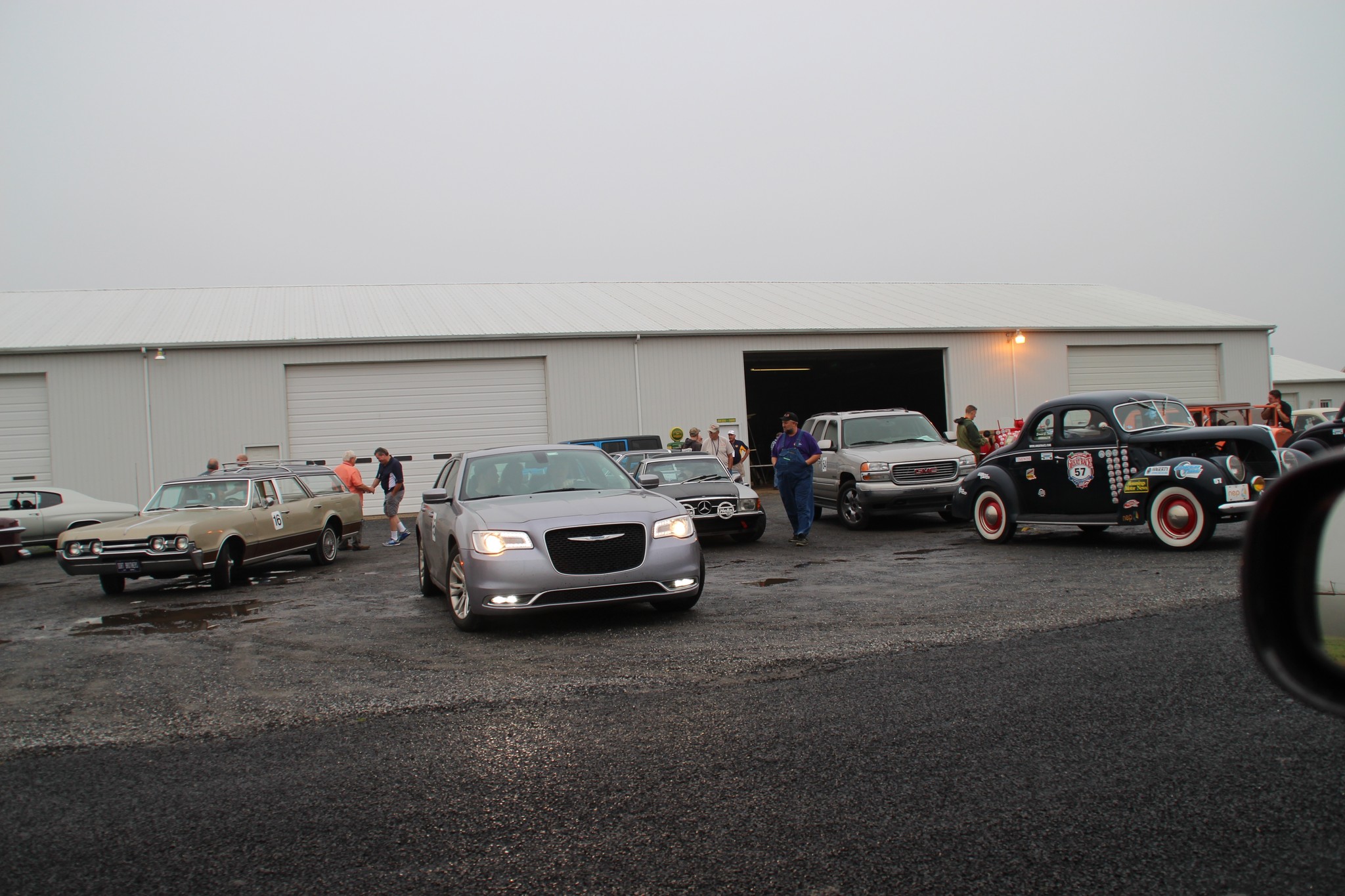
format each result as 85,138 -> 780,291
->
788,535 -> 798,542
796,537 -> 809,546
352,545 -> 369,551
338,545 -> 352,550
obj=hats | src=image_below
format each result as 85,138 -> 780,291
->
689,427 -> 700,436
727,430 -> 735,435
707,424 -> 720,433
777,433 -> 782,437
779,412 -> 799,423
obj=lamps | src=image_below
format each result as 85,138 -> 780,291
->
1013,330 -> 1026,344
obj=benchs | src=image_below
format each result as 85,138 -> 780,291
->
267,493 -> 309,501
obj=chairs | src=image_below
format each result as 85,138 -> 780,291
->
7,499 -> 34,510
649,472 -> 668,484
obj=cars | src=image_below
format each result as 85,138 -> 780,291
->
573,449 -> 681,487
1119,400 -> 1294,457
0,488 -> 140,551
1284,401 -> 1345,459
56,456 -> 362,596
949,391 -> 1314,551
415,444 -> 710,630
1289,408 -> 1342,437
633,455 -> 767,547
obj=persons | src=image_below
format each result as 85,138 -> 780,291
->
331,451 -> 375,550
681,424 -> 750,484
954,405 -> 989,465
225,453 -> 251,498
471,463 -> 583,498
770,413 -> 823,545
1260,390 -> 1294,434
193,458 -> 228,500
366,448 -> 411,546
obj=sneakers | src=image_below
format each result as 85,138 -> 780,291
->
382,539 -> 400,546
398,529 -> 411,542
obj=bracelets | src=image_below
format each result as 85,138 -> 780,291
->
1277,410 -> 1281,411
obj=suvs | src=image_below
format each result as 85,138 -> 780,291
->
804,408 -> 978,530
554,435 -> 663,475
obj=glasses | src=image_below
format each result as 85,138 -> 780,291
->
1268,397 -> 1276,400
971,408 -> 977,411
